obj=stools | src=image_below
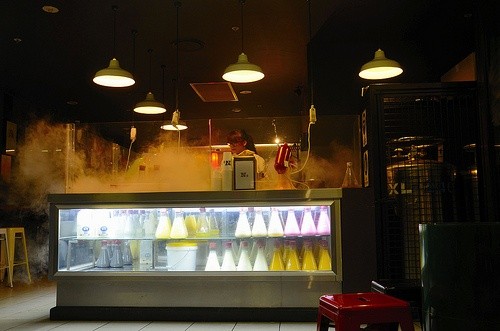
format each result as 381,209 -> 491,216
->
317,292 -> 415,331
0,227 -> 31,287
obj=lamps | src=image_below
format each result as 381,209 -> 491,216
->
93,7 -> 135,88
133,49 -> 167,114
359,48 -> 403,80
161,79 -> 188,131
222,0 -> 265,83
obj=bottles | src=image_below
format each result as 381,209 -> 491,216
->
233,206 -> 331,272
104,210 -> 219,239
204,242 -> 221,271
96,240 -> 133,267
221,243 -> 235,271
342,162 -> 359,188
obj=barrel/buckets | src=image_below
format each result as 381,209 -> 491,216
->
180,240 -> 208,265
165,241 -> 198,272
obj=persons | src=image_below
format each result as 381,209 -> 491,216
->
220,129 -> 265,180
